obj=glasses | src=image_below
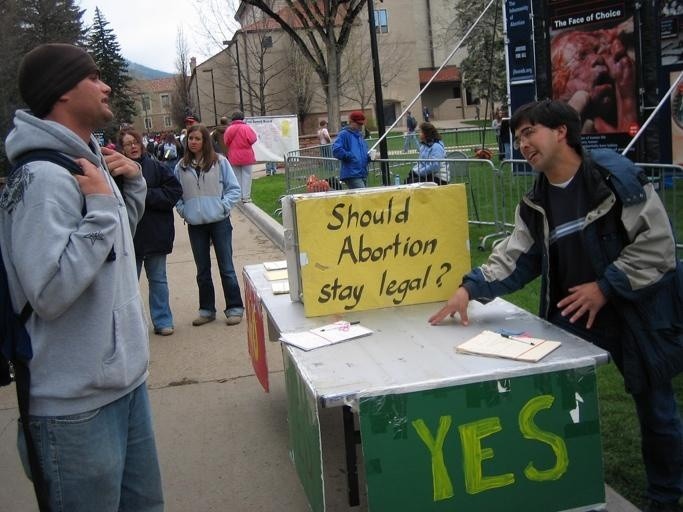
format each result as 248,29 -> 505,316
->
513,125 -> 543,150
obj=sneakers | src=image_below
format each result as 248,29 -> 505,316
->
193,316 -> 216,325
227,315 -> 242,324
240,197 -> 252,202
155,327 -> 173,335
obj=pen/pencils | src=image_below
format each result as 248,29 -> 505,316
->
501,333 -> 534,346
321,321 -> 360,331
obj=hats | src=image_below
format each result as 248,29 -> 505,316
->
18,43 -> 100,119
185,113 -> 200,122
350,112 -> 366,124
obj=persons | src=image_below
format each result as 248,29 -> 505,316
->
549,31 -> 638,133
401,111 -> 420,152
318,120 -> 333,169
474,109 -> 513,162
423,104 -> 430,121
1,44 -> 163,512
332,112 -> 372,189
224,110 -> 257,203
110,127 -> 183,335
266,161 -> 277,177
428,100 -> 683,510
404,123 -> 451,185
91,115 -> 231,175
173,124 -> 241,326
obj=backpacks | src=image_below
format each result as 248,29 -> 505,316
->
406,117 -> 416,128
0,151 -> 85,387
161,142 -> 178,161
147,140 -> 156,155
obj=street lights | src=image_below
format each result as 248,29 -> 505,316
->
203,68 -> 218,127
223,41 -> 244,116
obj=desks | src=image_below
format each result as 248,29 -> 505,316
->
241,259 -> 612,511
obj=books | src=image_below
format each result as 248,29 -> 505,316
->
278,318 -> 376,351
457,328 -> 563,362
263,261 -> 293,295
